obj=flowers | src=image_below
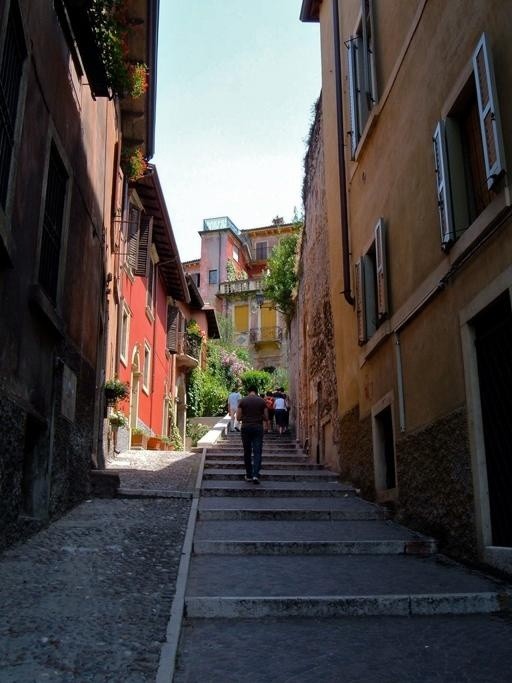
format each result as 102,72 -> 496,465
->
108,410 -> 128,431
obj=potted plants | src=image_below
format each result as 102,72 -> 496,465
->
104,379 -> 129,408
160,434 -> 168,450
130,424 -> 150,449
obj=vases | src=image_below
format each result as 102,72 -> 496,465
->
110,418 -> 118,424
168,441 -> 174,450
147,436 -> 160,449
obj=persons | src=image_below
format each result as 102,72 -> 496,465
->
235,383 -> 269,483
226,385 -> 292,436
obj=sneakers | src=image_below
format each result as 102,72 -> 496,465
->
230,429 -> 234,432
244,475 -> 252,482
252,476 -> 261,484
234,426 -> 241,432
263,428 -> 288,436
